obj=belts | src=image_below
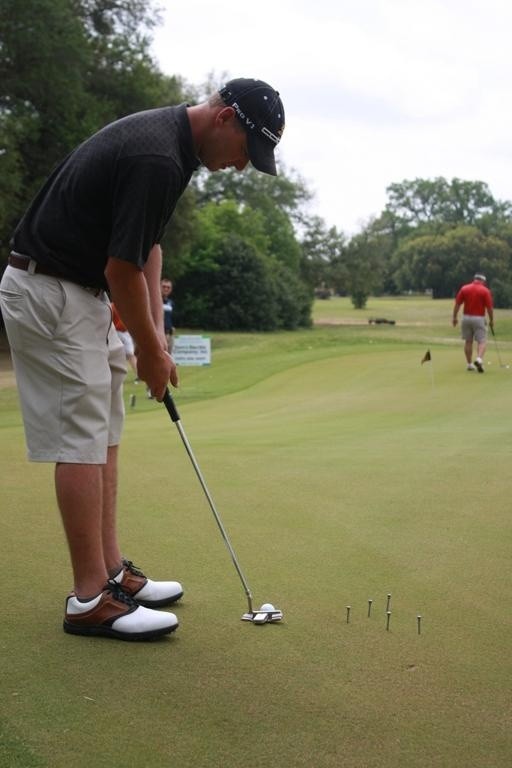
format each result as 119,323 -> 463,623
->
6,253 -> 108,291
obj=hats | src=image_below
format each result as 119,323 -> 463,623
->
474,272 -> 486,281
218,78 -> 285,176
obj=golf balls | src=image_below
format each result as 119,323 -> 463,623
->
260,602 -> 274,611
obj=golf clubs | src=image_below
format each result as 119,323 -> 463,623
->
489,325 -> 509,368
162,387 -> 283,624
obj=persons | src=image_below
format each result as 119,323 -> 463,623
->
452,273 -> 495,373
0,76 -> 284,641
109,301 -> 140,384
146,278 -> 175,398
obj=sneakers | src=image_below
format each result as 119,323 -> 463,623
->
63,578 -> 178,642
468,357 -> 484,372
108,564 -> 182,606
